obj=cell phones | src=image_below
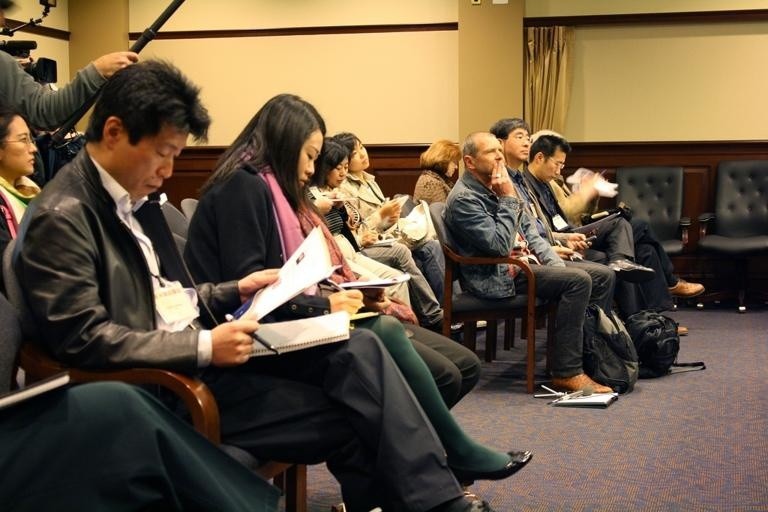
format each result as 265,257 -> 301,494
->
584,236 -> 597,243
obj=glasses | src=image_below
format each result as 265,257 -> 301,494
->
543,156 -> 566,170
0,138 -> 37,146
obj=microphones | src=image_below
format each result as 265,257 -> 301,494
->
546,387 -> 592,406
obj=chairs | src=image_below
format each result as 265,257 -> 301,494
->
615,166 -> 693,311
698,160 -> 768,314
428,201 -> 540,393
3,240 -> 308,510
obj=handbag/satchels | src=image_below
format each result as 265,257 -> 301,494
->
622,306 -> 680,379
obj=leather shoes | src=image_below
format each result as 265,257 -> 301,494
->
675,325 -> 688,337
549,372 -> 614,395
669,278 -> 703,299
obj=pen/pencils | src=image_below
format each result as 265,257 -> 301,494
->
224,313 -> 280,358
554,239 -> 575,262
583,234 -> 598,243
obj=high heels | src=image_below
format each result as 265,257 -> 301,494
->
450,448 -> 534,487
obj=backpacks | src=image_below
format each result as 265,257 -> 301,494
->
583,302 -> 639,397
